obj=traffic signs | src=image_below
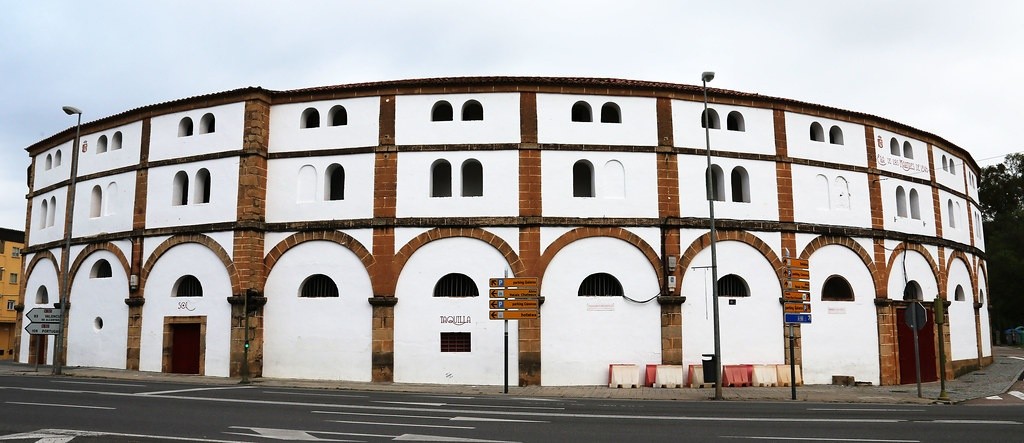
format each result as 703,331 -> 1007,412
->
24,309 -> 60,335
782,257 -> 811,323
489,277 -> 538,320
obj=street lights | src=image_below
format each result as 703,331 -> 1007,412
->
53,106 -> 83,375
702,72 -> 723,400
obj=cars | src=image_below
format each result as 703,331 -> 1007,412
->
1005,326 -> 1024,336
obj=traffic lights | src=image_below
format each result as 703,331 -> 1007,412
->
247,289 -> 267,314
245,340 -> 249,348
228,297 -> 245,307
